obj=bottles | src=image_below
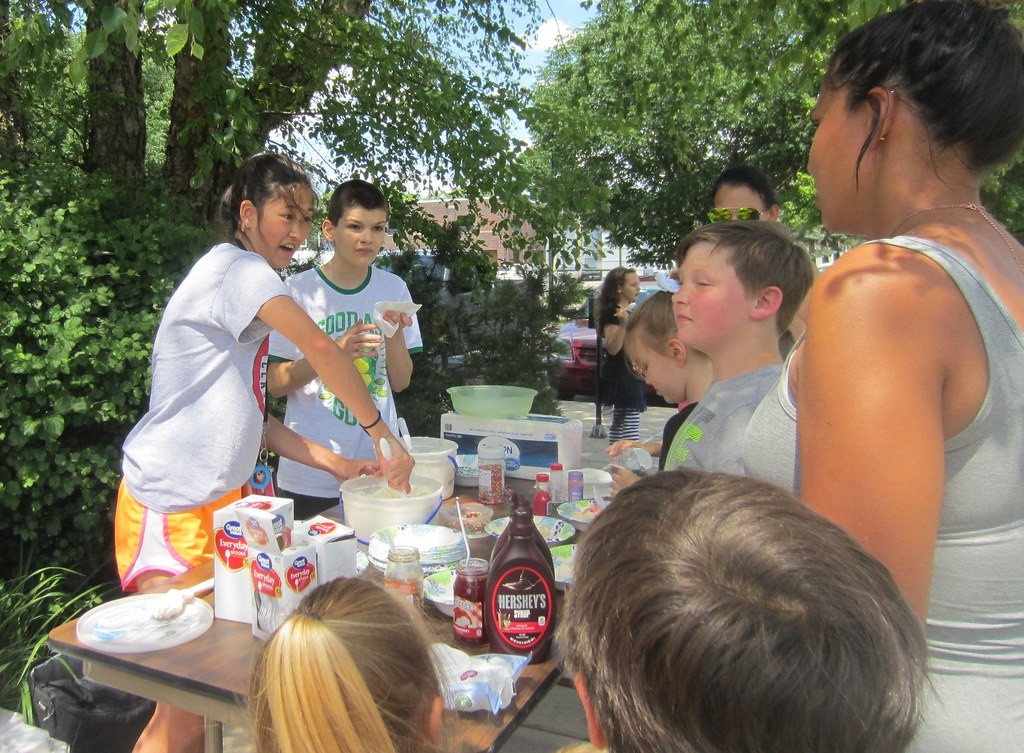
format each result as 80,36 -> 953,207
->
550,464 -> 565,503
532,474 -> 552,517
568,471 -> 583,501
489,478 -> 555,582
477,446 -> 507,505
384,545 -> 424,616
453,558 -> 490,646
485,508 -> 557,664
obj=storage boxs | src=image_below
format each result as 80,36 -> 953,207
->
212,494 -> 357,644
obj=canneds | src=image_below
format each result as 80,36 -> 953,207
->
384,545 -> 424,620
452,558 -> 492,648
478,446 -> 506,504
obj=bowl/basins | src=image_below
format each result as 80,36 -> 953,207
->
454,455 -> 478,487
438,502 -> 493,539
367,523 -> 470,583
446,385 -> 538,418
556,500 -> 610,532
484,515 -> 575,548
422,570 -> 457,617
550,545 -> 579,590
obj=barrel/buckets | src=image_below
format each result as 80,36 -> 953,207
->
397,436 -> 459,500
338,473 -> 444,549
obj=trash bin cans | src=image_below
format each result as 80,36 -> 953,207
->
28,654 -> 158,753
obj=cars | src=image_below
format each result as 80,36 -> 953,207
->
547,281 -> 678,408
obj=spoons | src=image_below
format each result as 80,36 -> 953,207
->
614,303 -> 636,316
258,581 -> 261,591
225,550 -> 231,568
295,579 -> 299,592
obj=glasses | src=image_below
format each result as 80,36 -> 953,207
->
707,206 -> 765,223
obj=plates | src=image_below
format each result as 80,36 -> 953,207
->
357,549 -> 369,576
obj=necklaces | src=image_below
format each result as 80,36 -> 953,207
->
888,203 -> 1022,272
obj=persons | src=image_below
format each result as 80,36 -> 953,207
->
268,179 -> 426,520
592,267 -> 647,447
251,576 -> 456,753
550,469 -> 947,753
604,165 -> 818,497
112,150 -> 415,753
786,0 -> 1024,753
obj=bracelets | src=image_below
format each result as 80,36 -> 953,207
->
360,410 -> 382,436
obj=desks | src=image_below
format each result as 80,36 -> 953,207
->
46,476 -> 564,753
